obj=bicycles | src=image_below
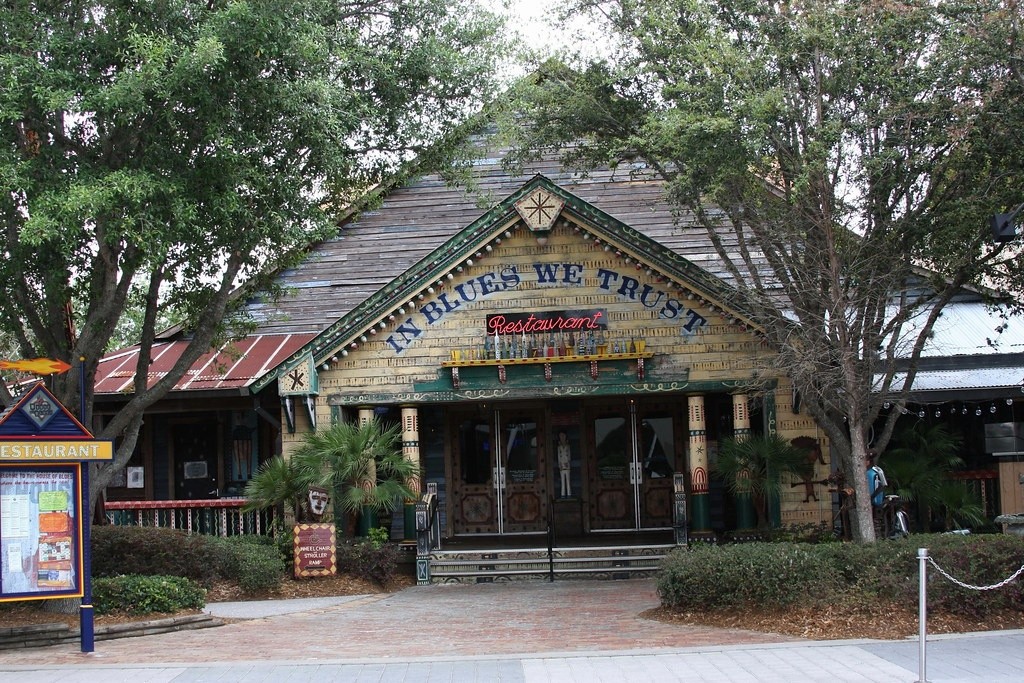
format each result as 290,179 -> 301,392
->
883,494 -> 910,538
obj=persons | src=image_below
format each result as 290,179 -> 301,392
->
865,449 -> 888,539
